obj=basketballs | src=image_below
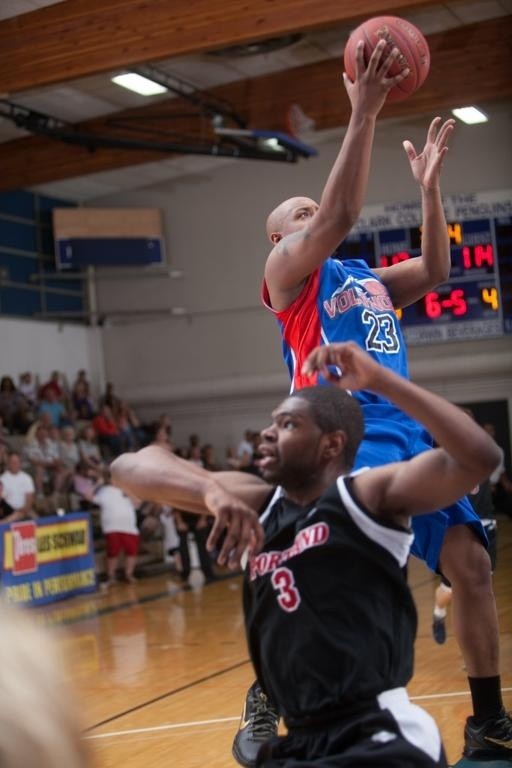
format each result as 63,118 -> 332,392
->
344,16 -> 430,103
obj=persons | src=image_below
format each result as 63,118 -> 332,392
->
110,340 -> 501,768
432,423 -> 503,643
233,37 -> 512,768
1,372 -> 262,588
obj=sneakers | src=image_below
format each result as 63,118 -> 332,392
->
233,679 -> 281,766
462,709 -> 512,763
433,610 -> 447,645
